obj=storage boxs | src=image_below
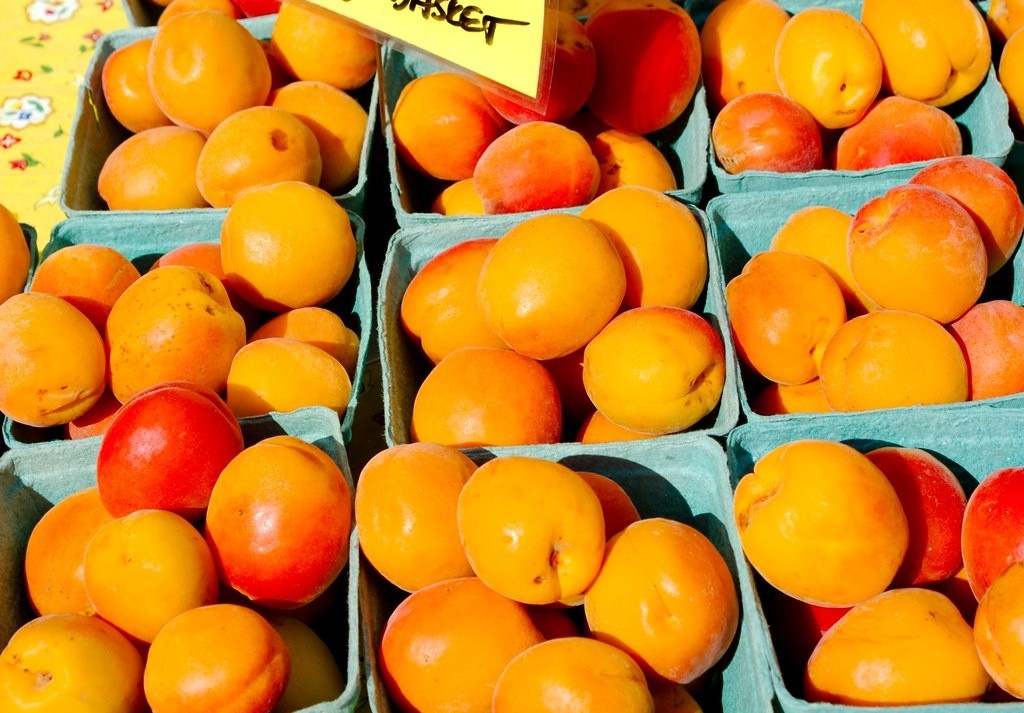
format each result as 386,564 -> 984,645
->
0,0 -> 1024,713
